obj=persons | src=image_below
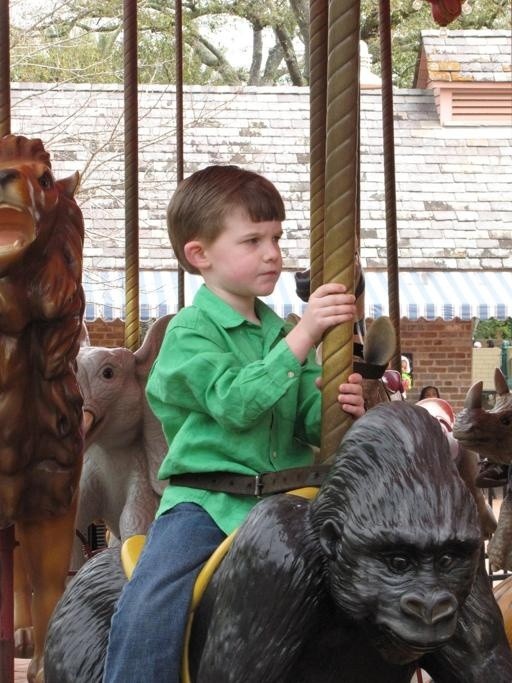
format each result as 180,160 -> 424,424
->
402,354 -> 412,401
104,161 -> 369,681
419,384 -> 439,399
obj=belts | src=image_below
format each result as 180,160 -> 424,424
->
169,465 -> 332,501
